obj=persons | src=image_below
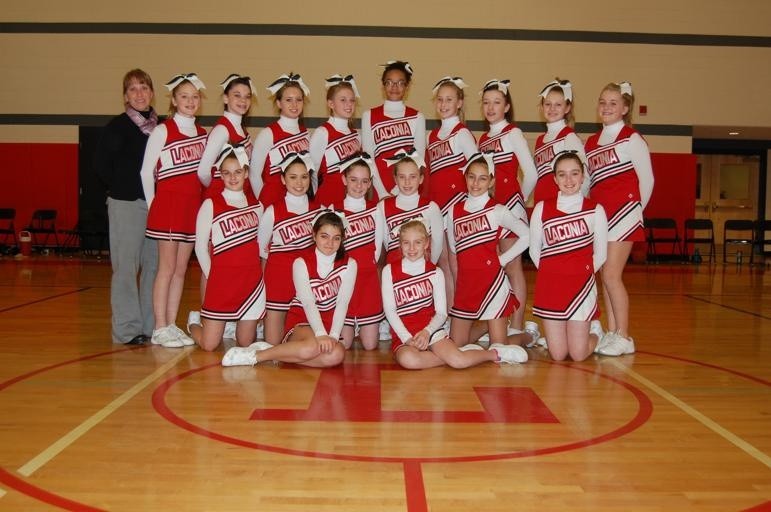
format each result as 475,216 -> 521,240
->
361,60 -> 429,211
328,151 -> 386,351
248,70 -> 313,207
378,146 -> 445,267
533,76 -> 590,209
93,68 -> 166,348
585,79 -> 658,358
184,142 -> 266,352
476,79 -> 540,343
196,72 -> 256,189
256,149 -> 328,347
528,148 -> 607,364
139,72 -> 209,348
422,75 -> 482,209
445,150 -> 541,349
221,203 -> 360,370
310,72 -> 365,210
381,215 -> 531,371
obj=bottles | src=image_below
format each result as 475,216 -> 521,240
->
693,248 -> 702,263
737,250 -> 743,263
19,231 -> 33,256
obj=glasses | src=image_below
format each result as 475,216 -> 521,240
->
385,78 -> 408,88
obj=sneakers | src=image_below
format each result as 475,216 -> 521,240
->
187,311 -> 202,335
249,341 -> 274,350
458,343 -> 484,352
151,327 -> 184,348
524,321 -> 540,348
222,347 -> 258,367
256,323 -> 264,340
125,333 -> 146,345
478,332 -> 489,342
222,321 -> 237,340
593,331 -> 615,353
536,337 -> 548,349
488,343 -> 528,364
169,323 -> 195,346
589,320 -> 605,342
377,320 -> 393,340
505,320 -> 524,337
599,328 -> 634,356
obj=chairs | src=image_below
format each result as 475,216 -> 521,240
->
635,218 -> 771,268
2,207 -> 106,259
